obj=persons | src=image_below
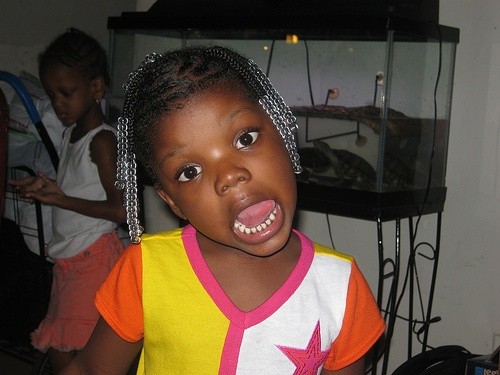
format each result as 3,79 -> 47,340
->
7,26 -> 119,375
52,46 -> 386,374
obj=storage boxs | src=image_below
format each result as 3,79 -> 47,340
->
103,0 -> 460,223
